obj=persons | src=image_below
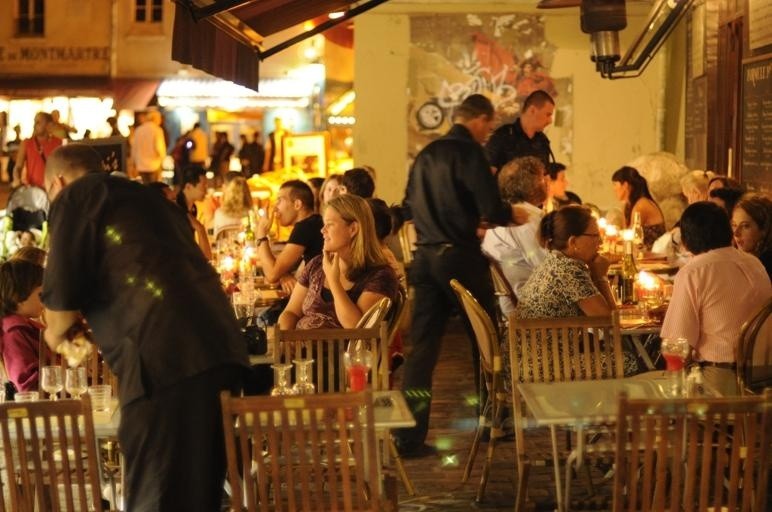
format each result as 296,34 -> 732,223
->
0,90 -> 772,512
503,60 -> 558,103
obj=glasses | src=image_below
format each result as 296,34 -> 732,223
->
582,233 -> 601,241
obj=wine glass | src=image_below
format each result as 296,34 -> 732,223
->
41,366 -> 63,402
291,358 -> 317,395
65,367 -> 88,401
269,363 -> 295,396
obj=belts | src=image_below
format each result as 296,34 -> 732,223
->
698,361 -> 737,369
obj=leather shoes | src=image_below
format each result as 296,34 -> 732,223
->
479,426 -> 515,442
393,439 -> 437,458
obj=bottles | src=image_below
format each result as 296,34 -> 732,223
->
685,366 -> 706,416
620,239 -> 640,306
632,210 -> 645,248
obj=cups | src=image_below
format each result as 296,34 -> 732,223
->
661,336 -> 690,376
87,384 -> 112,416
232,290 -> 256,327
343,349 -> 373,393
12,392 -> 40,402
